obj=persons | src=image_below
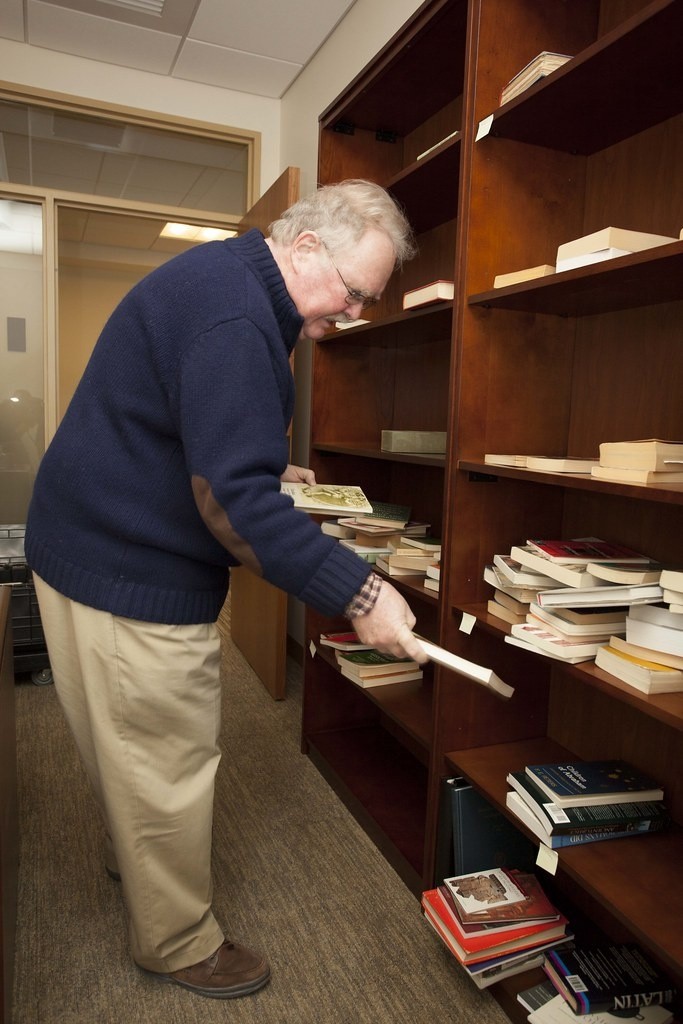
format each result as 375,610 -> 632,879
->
24,178 -> 414,998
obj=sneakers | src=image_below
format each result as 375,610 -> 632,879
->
106,862 -> 122,881
133,939 -> 270,998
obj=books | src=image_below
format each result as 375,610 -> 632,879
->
492,264 -> 556,290
332,651 -> 424,690
527,756 -> 663,809
320,499 -> 441,593
420,775 -> 683,1024
316,628 -> 384,652
417,131 -> 459,161
402,280 -> 455,313
553,227 -> 683,275
481,537 -> 683,694
277,479 -> 376,518
485,438 -> 683,490
501,51 -> 574,107
410,628 -> 515,699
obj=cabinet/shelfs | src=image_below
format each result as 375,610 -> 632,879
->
299,0 -> 683,1024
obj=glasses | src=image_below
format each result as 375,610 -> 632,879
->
299,228 -> 376,311
